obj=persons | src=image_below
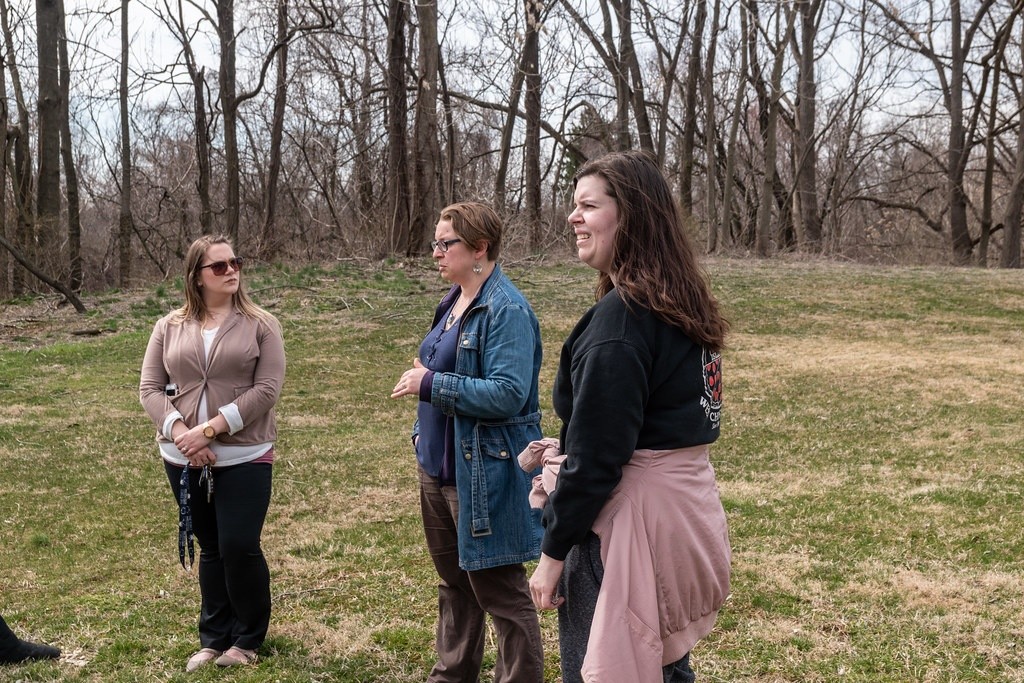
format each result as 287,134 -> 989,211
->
391,203 -> 545,683
140,235 -> 286,672
517,153 -> 731,683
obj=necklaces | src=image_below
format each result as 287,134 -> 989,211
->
447,297 -> 461,325
213,309 -> 230,319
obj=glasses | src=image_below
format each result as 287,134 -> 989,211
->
431,238 -> 461,252
199,257 -> 244,276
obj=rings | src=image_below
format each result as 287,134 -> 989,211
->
204,459 -> 208,462
182,448 -> 187,452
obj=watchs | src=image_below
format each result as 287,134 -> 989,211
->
203,422 -> 217,440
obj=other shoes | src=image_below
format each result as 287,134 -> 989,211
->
0,638 -> 60,662
215,646 -> 259,666
186,647 -> 223,672
